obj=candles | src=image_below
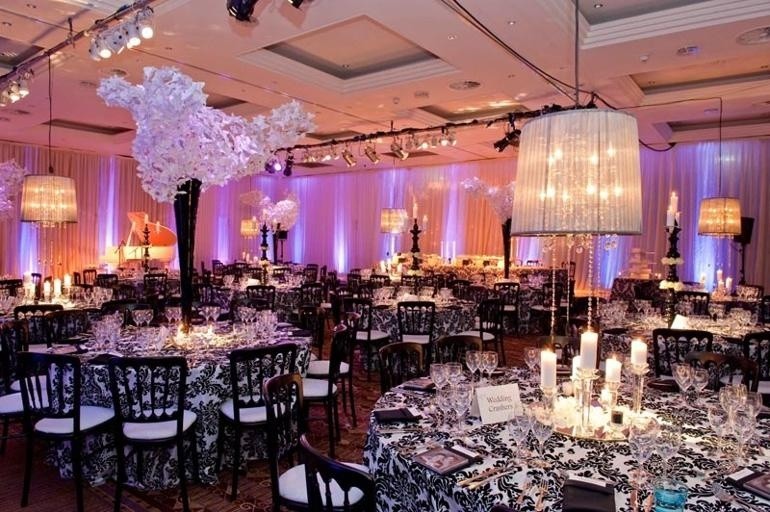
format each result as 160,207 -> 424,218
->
666,191 -> 681,227
423,215 -> 427,229
251,208 -> 278,230
413,206 -> 418,219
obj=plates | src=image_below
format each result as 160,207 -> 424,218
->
529,400 -> 658,443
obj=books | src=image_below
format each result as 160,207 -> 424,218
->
412,445 -> 470,475
742,473 -> 770,500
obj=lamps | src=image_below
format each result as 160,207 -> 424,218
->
265,102 -> 599,176
380,157 -> 408,234
698,99 -> 743,241
224,0 -> 260,24
510,0 -> 646,239
239,178 -> 260,242
20,57 -> 79,231
1,0 -> 154,110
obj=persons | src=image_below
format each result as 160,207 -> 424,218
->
763,477 -> 770,483
419,448 -> 445,472
436,453 -> 457,473
761,482 -> 770,489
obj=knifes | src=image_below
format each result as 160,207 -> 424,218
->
456,467 -> 505,487
467,471 -> 513,492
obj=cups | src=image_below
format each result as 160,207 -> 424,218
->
654,479 -> 689,512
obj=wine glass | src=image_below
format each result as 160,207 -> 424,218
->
0,287 -> 25,317
628,416 -> 657,484
270,273 -> 309,291
708,382 -> 762,465
670,361 -> 708,410
82,286 -> 114,310
537,356 -> 649,440
705,303 -> 759,333
370,285 -> 455,306
654,417 -> 682,488
429,351 -> 499,431
89,304 -> 278,352
523,346 -> 554,381
528,402 -> 555,468
505,402 -> 532,466
599,298 -> 663,332
221,273 -> 254,295
674,299 -> 693,321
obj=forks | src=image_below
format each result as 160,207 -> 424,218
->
535,479 -> 549,512
516,480 -> 532,505
712,486 -> 757,512
